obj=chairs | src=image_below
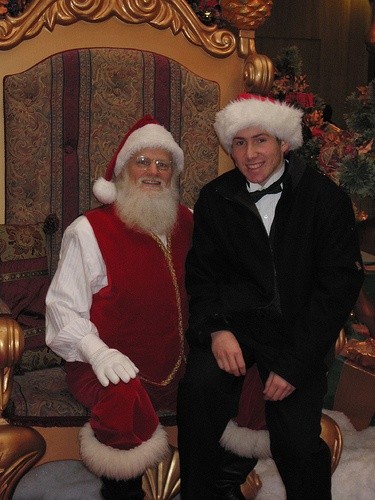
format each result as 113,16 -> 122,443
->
0,0 -> 344,500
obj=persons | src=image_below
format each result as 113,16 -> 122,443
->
178,94 -> 365,500
44,111 -> 195,500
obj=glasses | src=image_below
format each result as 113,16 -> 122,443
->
131,156 -> 174,170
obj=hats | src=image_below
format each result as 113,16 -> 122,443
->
212,93 -> 304,154
220,364 -> 272,459
93,112 -> 184,204
79,370 -> 171,481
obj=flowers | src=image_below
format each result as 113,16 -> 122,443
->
271,75 -> 373,221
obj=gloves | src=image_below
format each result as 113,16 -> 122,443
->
88,345 -> 139,387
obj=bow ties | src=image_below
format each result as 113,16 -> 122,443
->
248,181 -> 282,203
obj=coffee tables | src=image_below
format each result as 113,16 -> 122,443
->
333,225 -> 375,431
